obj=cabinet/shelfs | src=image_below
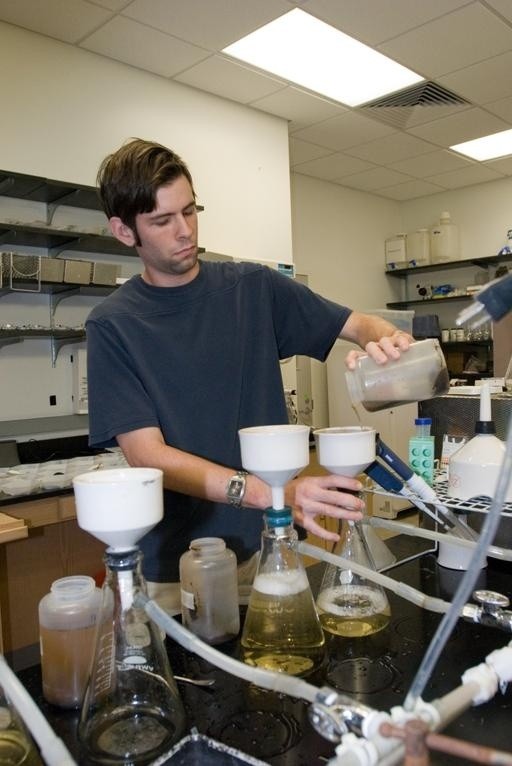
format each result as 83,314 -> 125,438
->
384,253 -> 512,381
0,169 -> 207,367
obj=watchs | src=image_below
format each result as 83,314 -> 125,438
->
224,470 -> 251,512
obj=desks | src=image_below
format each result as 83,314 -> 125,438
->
0,530 -> 511,766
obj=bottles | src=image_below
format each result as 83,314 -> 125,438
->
442,327 -> 465,343
316,487 -> 393,639
344,337 -> 451,413
76,546 -> 188,766
178,535 -> 242,646
408,417 -> 436,487
38,574 -> 116,712
240,485 -> 329,680
384,211 -> 461,272
447,419 -> 512,503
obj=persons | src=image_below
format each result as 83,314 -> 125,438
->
81,137 -> 424,605
